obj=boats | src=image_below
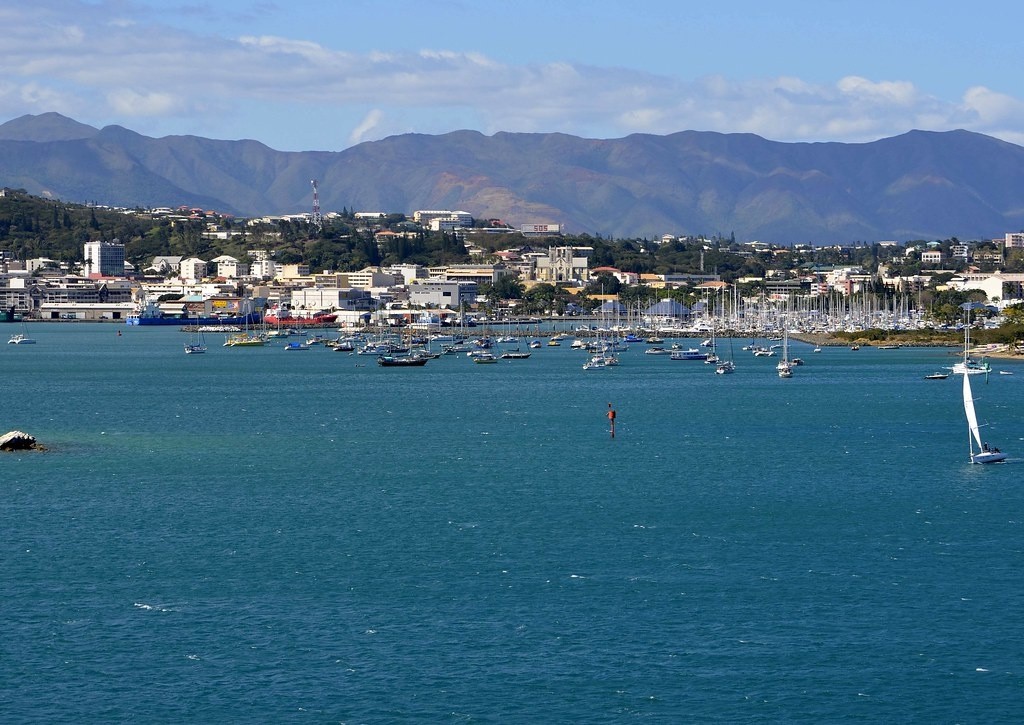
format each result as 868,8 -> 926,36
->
266,303 -> 338,327
944,359 -> 993,376
771,343 -> 791,349
743,343 -> 760,351
812,347 -> 822,354
0,425 -> 40,451
646,346 -> 672,354
877,346 -> 903,351
10,333 -> 38,349
645,336 -> 666,344
851,343 -> 861,352
925,370 -> 950,380
670,348 -> 711,359
754,349 -> 777,358
777,358 -> 804,381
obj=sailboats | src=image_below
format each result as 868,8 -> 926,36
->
714,288 -> 737,375
178,319 -> 646,370
960,365 -> 1008,465
705,292 -> 719,364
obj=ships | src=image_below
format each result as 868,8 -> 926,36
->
125,299 -> 262,327
